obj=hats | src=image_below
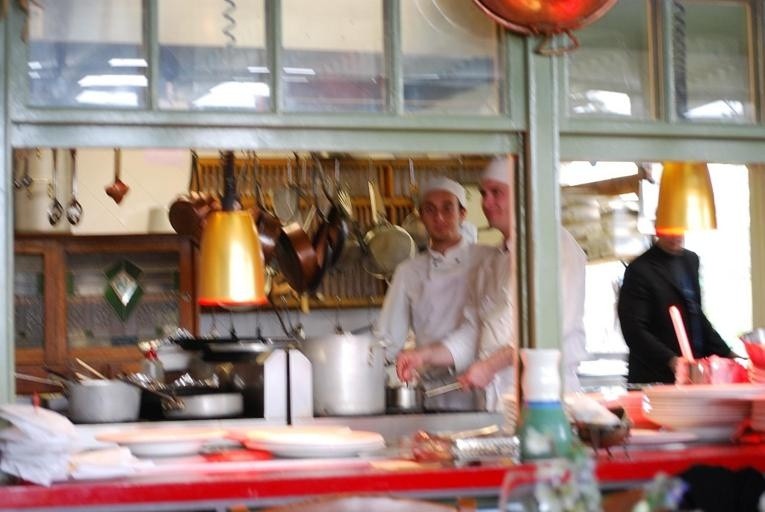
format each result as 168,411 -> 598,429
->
420,176 -> 468,209
479,155 -> 512,188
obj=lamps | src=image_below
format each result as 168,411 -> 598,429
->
656,162 -> 717,237
197,151 -> 267,308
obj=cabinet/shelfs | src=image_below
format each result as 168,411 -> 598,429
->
1,404 -> 763,512
14,235 -> 193,395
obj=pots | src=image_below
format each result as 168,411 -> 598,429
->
168,156 -> 201,234
360,159 -> 416,288
252,159 -> 280,266
13,372 -> 141,422
312,157 -> 345,274
402,157 -> 428,246
280,163 -> 316,295
472,0 -> 618,56
119,372 -> 243,418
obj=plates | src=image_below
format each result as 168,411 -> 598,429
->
116,431 -> 223,457
244,428 -> 388,461
642,385 -> 751,430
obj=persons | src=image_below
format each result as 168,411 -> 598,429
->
617,234 -> 735,391
396,159 -> 588,413
374,177 -> 493,412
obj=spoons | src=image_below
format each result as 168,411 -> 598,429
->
49,149 -> 63,227
67,149 -> 83,225
